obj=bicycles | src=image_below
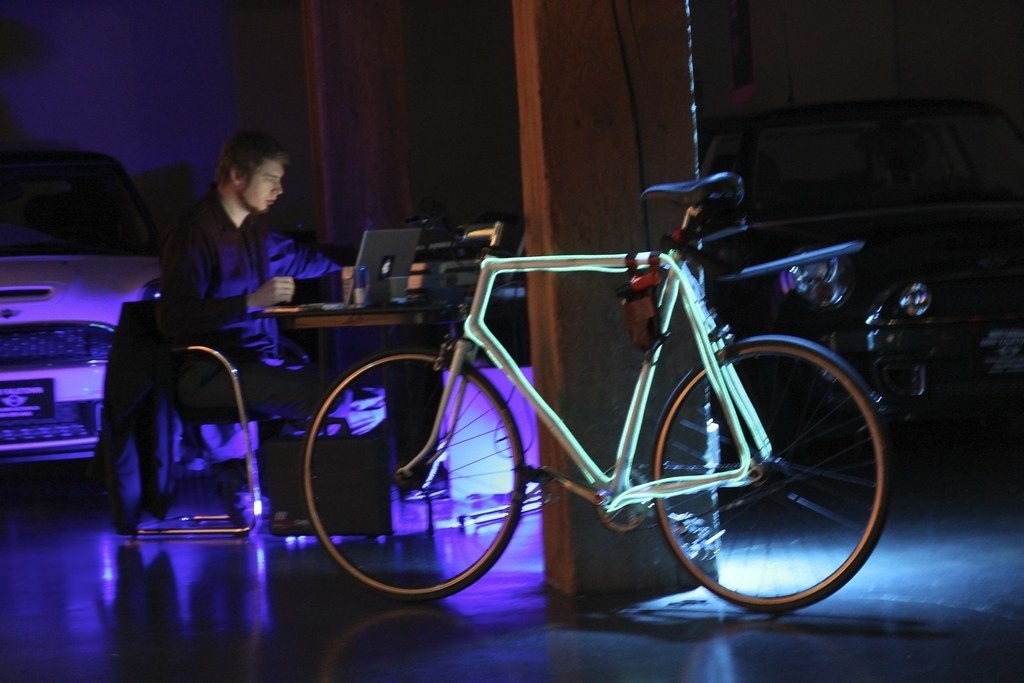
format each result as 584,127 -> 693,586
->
300,170 -> 892,615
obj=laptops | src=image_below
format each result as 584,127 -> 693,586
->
298,227 -> 422,311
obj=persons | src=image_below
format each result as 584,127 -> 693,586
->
154,127 -> 351,535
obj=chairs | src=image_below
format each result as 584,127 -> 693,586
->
711,152 -> 774,225
865,127 -> 929,204
97,278 -> 259,539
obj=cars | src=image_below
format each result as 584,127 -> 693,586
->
701,94 -> 1023,450
0,146 -> 169,464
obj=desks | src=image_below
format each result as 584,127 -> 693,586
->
250,301 -> 526,503
